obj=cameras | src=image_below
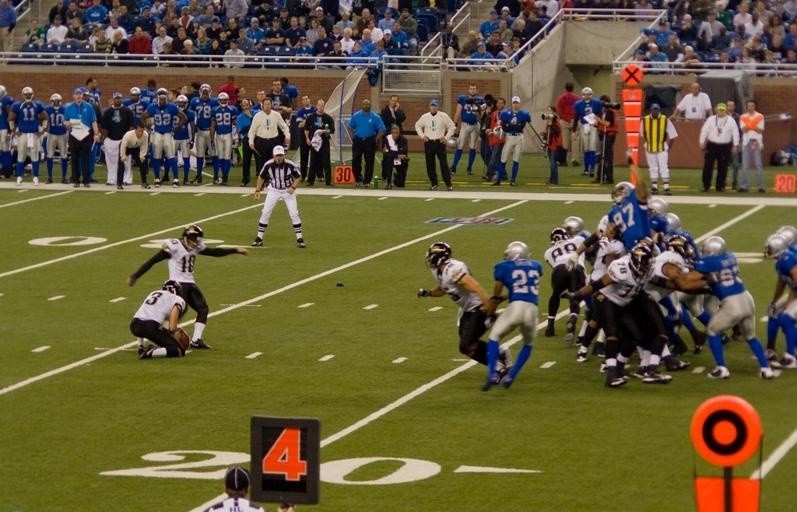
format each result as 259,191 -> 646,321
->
542,113 -> 556,119
599,101 -> 620,110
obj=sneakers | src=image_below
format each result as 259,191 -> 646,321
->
138,345 -> 144,354
511,180 -> 517,186
491,182 -> 500,186
355,182 -> 362,187
251,237 -> 264,246
363,183 -> 370,187
189,338 -> 214,350
447,185 -> 453,191
431,185 -> 439,190
545,327 -> 797,388
478,345 -> 513,392
385,184 -> 393,189
16,175 -> 229,189
296,237 -> 305,247
139,344 -> 156,360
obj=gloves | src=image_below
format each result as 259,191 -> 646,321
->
559,288 -> 578,301
417,287 -> 432,298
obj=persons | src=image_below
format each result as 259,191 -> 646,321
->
205,466 -> 294,512
640,83 -> 767,196
345,83 -> 532,190
544,183 -> 797,387
559,1 -> 797,78
543,82 -> 617,186
126,224 -> 248,360
0,74 -> 335,190
252,145 -> 305,248
417,241 -> 544,392
1,0 -> 561,72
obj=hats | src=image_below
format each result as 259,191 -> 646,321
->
273,145 -> 285,155
430,98 -> 439,106
72,87 -> 83,95
224,467 -> 251,492
489,7 -> 497,15
112,92 -> 122,100
384,28 -> 392,36
716,103 -> 727,111
511,96 -> 521,104
476,41 -> 485,48
650,103 -> 660,110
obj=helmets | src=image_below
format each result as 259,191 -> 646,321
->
0,85 -> 7,100
549,228 -> 568,244
562,214 -> 584,236
22,87 -> 34,103
182,224 -> 204,249
424,242 -> 452,269
762,233 -> 789,259
775,225 -> 797,246
503,241 -> 528,260
581,86 -> 594,101
162,280 -> 184,297
129,83 -> 229,110
603,180 -> 727,274
49,93 -> 63,109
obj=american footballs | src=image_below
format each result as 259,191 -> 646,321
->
176,328 -> 189,349
